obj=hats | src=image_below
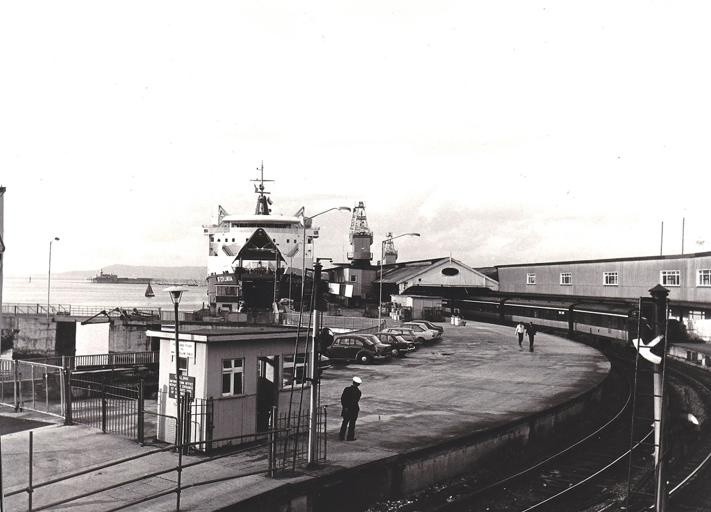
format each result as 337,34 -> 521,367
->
352,377 -> 362,384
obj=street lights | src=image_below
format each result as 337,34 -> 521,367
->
377,233 -> 420,339
299,206 -> 351,312
162,285 -> 190,452
46,237 -> 59,321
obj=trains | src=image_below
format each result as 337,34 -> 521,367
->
443,293 -> 643,348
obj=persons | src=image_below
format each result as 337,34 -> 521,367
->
515,321 -> 525,347
525,322 -> 536,352
340,376 -> 363,441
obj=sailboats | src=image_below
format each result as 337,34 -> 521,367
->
145,283 -> 154,297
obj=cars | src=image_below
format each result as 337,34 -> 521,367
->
326,320 -> 444,364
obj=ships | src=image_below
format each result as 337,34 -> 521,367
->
92,269 -> 152,284
202,161 -> 320,322
